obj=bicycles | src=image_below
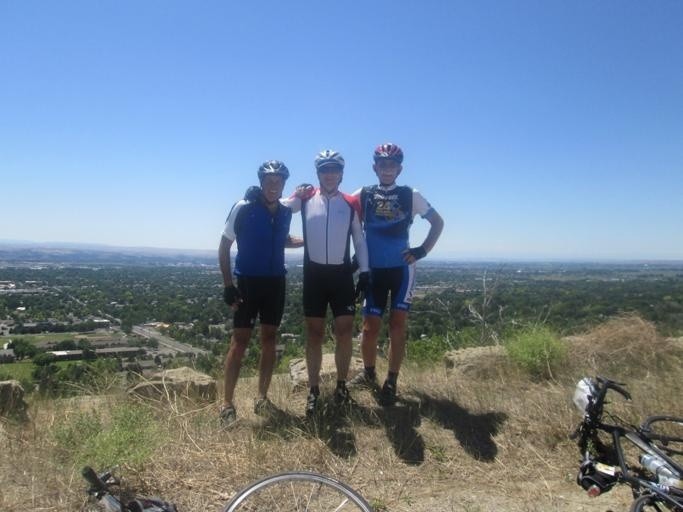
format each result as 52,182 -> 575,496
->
81,466 -> 372,512
569,375 -> 683,512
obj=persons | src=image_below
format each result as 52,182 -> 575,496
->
241,148 -> 374,420
215,159 -> 303,426
294,143 -> 444,406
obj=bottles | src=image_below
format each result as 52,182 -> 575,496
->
639,453 -> 683,480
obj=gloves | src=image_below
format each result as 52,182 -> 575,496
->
243,185 -> 262,203
222,286 -> 244,307
356,272 -> 373,304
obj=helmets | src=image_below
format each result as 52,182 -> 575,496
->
372,141 -> 405,165
257,159 -> 290,180
313,147 -> 346,170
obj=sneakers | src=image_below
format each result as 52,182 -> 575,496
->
345,368 -> 399,409
303,390 -> 319,418
330,386 -> 359,410
253,397 -> 286,420
219,405 -> 238,430
